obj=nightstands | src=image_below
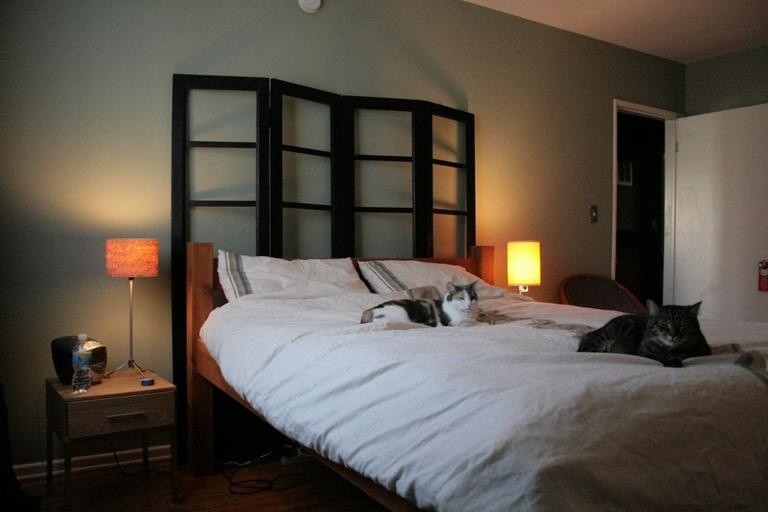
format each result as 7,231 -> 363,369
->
41,369 -> 183,507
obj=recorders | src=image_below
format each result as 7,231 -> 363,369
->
51,334 -> 107,384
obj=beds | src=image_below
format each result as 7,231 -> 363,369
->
179,239 -> 768,510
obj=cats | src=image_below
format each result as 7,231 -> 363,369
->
575,298 -> 712,368
359,279 -> 479,328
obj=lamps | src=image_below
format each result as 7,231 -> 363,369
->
105,237 -> 159,380
505,240 -> 541,298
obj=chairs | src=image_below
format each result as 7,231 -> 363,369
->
561,273 -> 648,319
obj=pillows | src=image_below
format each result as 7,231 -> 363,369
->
212,244 -> 505,313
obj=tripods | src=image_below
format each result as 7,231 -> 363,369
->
103,278 -> 149,378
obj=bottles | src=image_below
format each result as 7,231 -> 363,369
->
72,332 -> 93,391
